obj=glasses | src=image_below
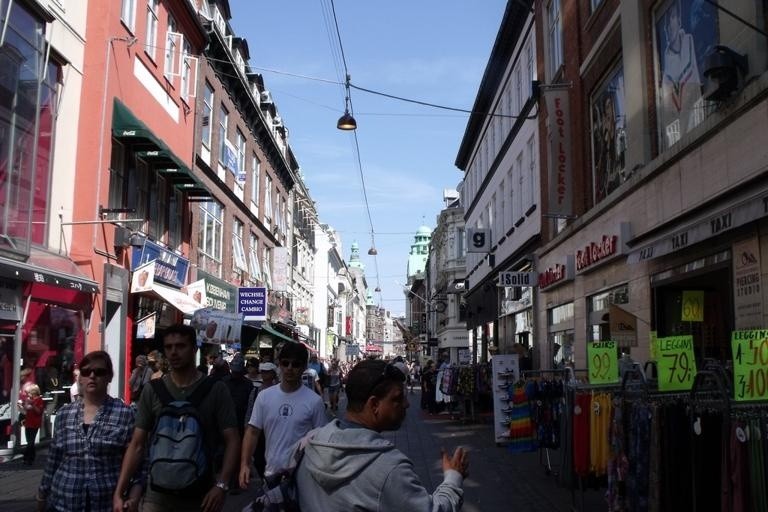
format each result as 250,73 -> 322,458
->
370,363 -> 394,392
281,360 -> 300,368
79,367 -> 110,377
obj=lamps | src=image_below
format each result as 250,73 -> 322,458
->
337,85 -> 357,131
368,231 -> 377,256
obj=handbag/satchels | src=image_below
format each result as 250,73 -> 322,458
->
242,437 -> 309,512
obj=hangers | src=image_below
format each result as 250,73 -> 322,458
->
695,389 -> 733,412
650,392 -> 690,405
732,402 -> 767,416
521,368 -> 572,384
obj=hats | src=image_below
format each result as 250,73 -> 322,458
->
212,356 -> 227,368
278,343 -> 308,362
260,362 -> 279,375
